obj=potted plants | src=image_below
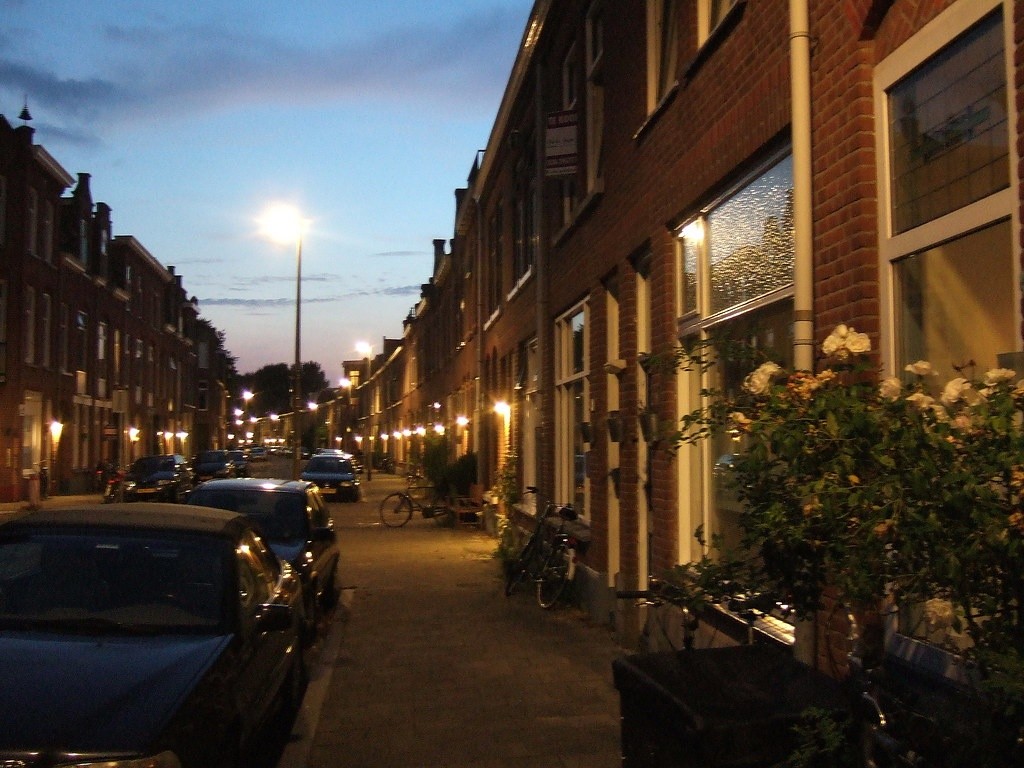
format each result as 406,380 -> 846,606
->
448,483 -> 459,506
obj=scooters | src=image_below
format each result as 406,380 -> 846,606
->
93,464 -> 123,503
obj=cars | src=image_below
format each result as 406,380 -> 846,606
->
194,450 -> 236,481
0,499 -> 309,768
178,476 -> 338,615
228,450 -> 251,477
300,454 -> 362,503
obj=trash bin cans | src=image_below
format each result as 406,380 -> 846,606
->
359,455 -> 367,465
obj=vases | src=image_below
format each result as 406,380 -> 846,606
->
460,498 -> 471,506
612,645 -> 867,768
445,496 -> 462,506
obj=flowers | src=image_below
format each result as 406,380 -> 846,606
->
637,309 -> 1024,768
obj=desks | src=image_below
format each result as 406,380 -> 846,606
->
448,506 -> 482,530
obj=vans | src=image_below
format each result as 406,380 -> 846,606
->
240,441 -> 363,472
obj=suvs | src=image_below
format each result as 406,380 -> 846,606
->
118,453 -> 190,505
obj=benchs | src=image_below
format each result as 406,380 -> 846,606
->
866,633 -> 1000,768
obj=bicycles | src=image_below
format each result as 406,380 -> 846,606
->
378,469 -> 452,528
33,459 -> 50,497
505,486 -> 582,612
